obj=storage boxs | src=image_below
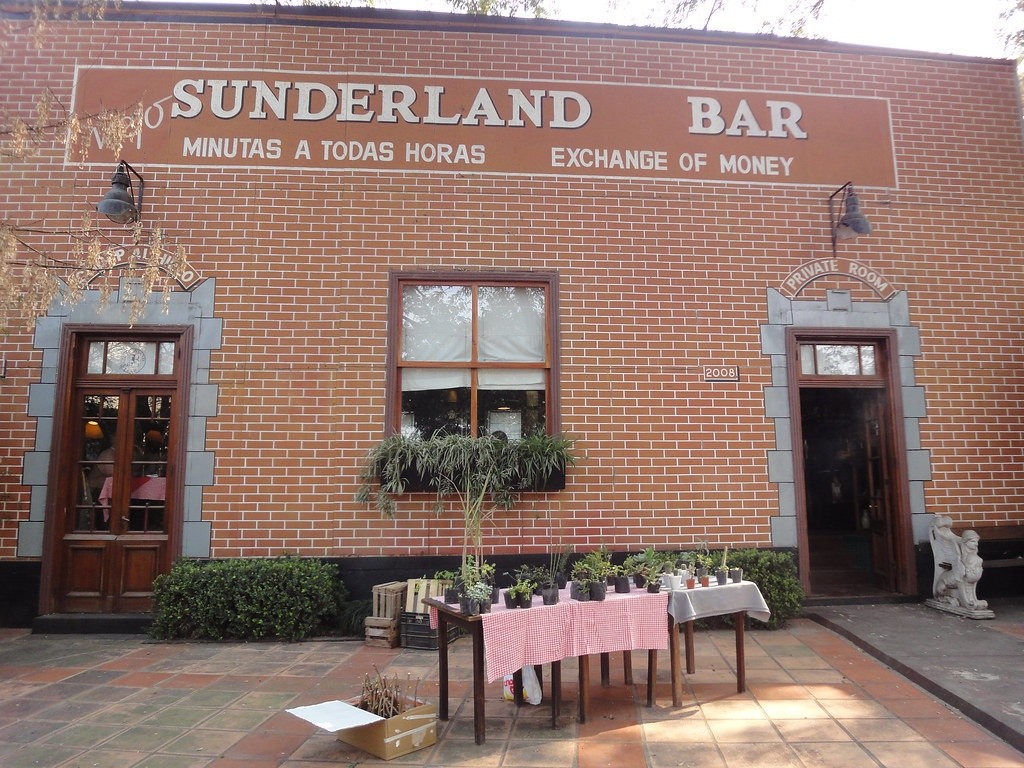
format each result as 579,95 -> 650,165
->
285,690 -> 437,760
365,578 -> 460,650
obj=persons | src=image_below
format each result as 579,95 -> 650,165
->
90,431 -> 167,487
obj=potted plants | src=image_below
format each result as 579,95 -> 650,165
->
570,538 -> 744,601
502,545 -> 575,608
434,555 -> 500,615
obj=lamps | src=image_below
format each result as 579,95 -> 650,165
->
830,181 -> 871,257
96,159 -> 144,225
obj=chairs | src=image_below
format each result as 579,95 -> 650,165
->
80,465 -> 100,526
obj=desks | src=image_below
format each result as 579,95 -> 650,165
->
100,475 -> 165,523
423,582 -> 669,746
623,575 -> 771,705
